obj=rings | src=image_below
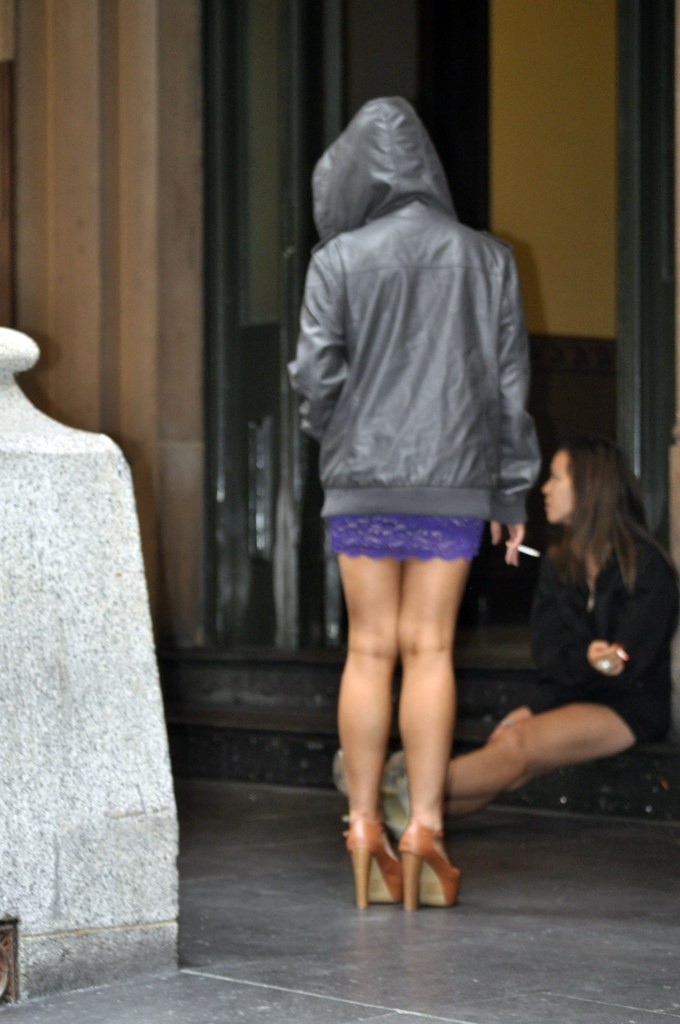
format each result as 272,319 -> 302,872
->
602,660 -> 609,667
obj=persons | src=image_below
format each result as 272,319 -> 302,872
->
287,96 -> 542,909
333,435 -> 680,844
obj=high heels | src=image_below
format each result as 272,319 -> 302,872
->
344,822 -> 403,909
398,825 -> 459,915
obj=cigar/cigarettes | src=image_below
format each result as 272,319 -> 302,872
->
505,541 -> 539,557
616,649 -> 628,660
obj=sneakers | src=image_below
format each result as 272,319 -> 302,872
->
334,749 -> 413,839
